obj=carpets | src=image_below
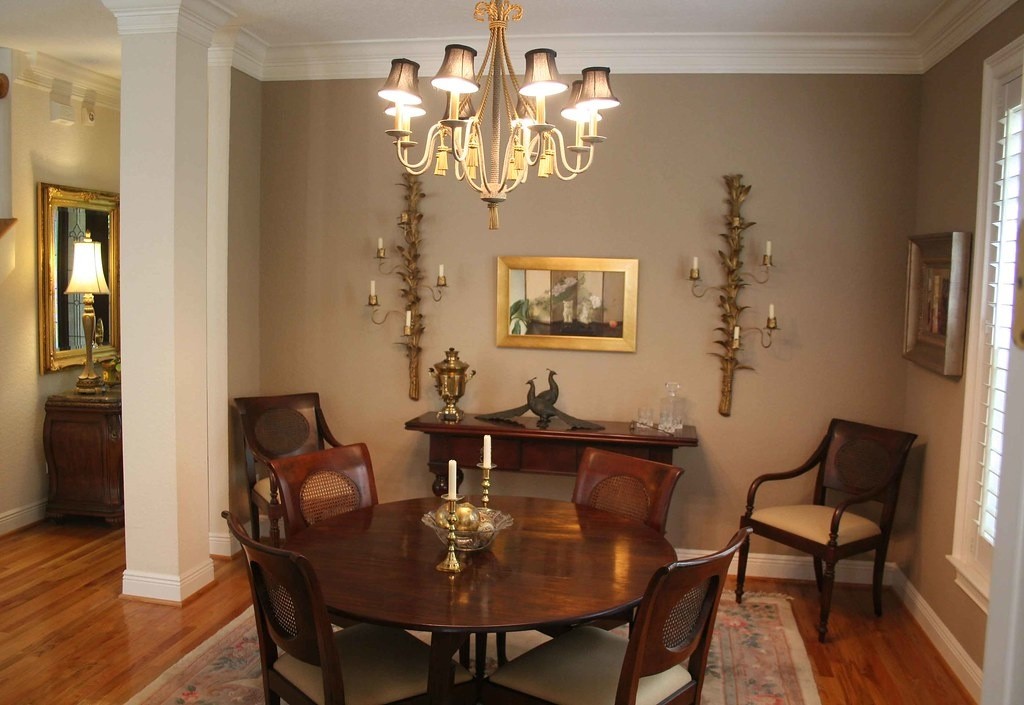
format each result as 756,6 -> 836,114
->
121,590 -> 822,705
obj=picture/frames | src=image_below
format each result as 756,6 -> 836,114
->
902,230 -> 973,377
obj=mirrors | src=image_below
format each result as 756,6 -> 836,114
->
37,180 -> 120,374
496,255 -> 640,352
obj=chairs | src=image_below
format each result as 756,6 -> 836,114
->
221,509 -> 475,705
496,447 -> 686,669
484,524 -> 753,705
268,443 -> 379,626
735,417 -> 918,642
233,392 -> 344,542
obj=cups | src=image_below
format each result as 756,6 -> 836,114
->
637,407 -> 653,428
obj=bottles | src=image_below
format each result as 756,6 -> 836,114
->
102,362 -> 116,385
660,384 -> 683,429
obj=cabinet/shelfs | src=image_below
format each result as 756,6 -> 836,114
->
42,387 -> 124,528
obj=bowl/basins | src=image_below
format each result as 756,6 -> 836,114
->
421,508 -> 514,551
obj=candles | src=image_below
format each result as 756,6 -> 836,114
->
483,435 -> 492,467
360,234 -> 776,340
448,459 -> 457,498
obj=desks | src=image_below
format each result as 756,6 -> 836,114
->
280,494 -> 677,705
405,410 -> 699,497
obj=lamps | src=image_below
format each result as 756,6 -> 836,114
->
376,0 -> 621,230
64,230 -> 110,395
